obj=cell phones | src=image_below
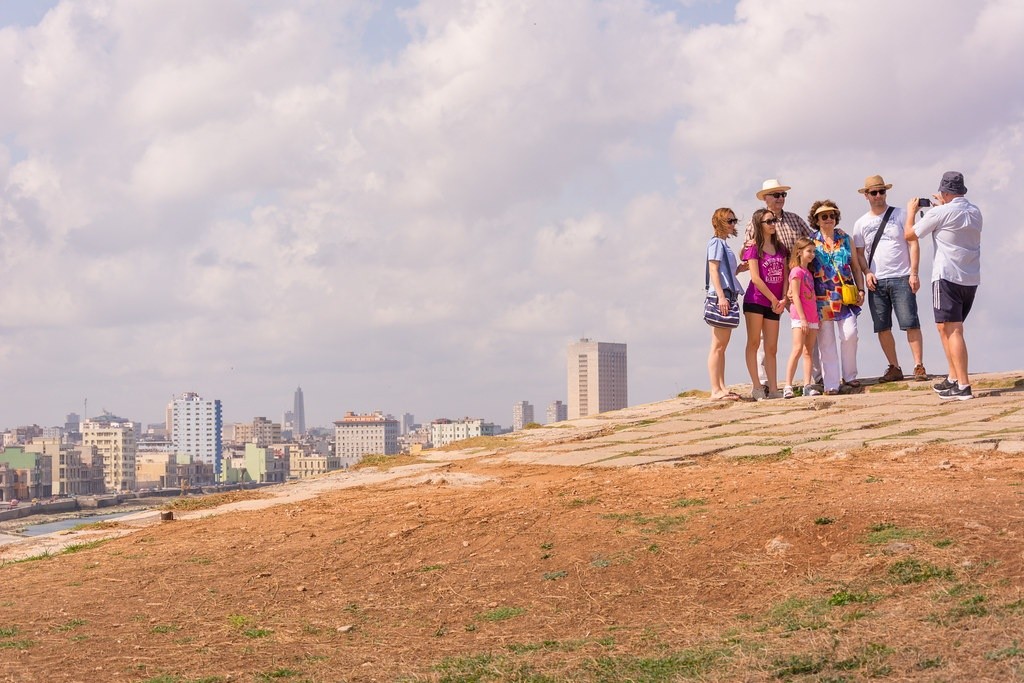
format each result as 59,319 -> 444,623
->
919,198 -> 931,207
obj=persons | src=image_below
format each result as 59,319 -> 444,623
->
853,174 -> 927,383
707,208 -> 750,402
787,200 -> 865,396
783,237 -> 820,398
739,178 -> 823,389
904,172 -> 983,400
742,209 -> 791,402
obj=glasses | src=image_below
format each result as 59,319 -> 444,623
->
868,189 -> 886,196
765,192 -> 787,198
822,214 -> 837,220
761,217 -> 777,225
727,218 -> 738,225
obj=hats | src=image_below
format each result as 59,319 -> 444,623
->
813,205 -> 839,217
938,171 -> 967,194
858,176 -> 892,194
756,180 -> 791,200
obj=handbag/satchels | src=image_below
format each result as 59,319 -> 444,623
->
842,284 -> 859,306
703,297 -> 740,329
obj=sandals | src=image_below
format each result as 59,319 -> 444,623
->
783,386 -> 795,398
803,387 -> 821,396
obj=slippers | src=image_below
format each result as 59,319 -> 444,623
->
712,392 -> 739,401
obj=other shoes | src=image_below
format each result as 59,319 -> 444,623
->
768,392 -> 783,399
828,389 -> 838,395
752,388 -> 767,402
842,379 -> 860,387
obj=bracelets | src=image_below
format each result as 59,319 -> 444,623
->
859,289 -> 865,294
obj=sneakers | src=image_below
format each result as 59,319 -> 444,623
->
933,379 -> 958,393
914,365 -> 928,381
938,380 -> 973,399
879,365 -> 904,383
